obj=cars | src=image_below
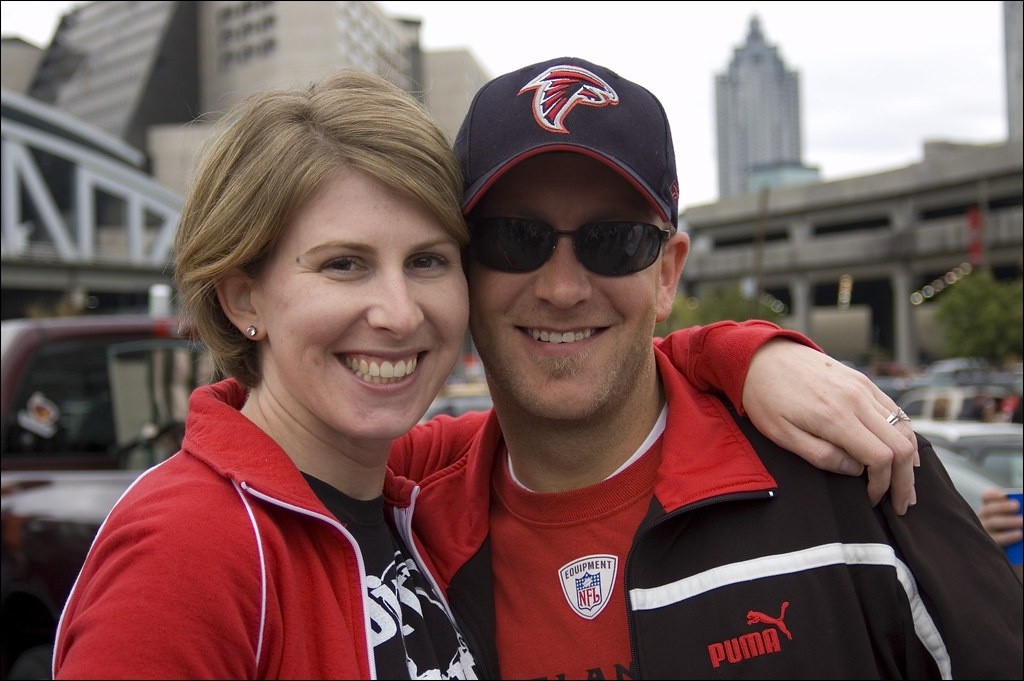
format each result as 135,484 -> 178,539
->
890,380 -> 1024,539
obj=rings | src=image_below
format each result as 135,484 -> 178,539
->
886,411 -> 900,426
897,406 -> 911,422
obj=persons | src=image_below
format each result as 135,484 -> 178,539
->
978,487 -> 1023,586
412,57 -> 1024,681
50,68 -> 921,681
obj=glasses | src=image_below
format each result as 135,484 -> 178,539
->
460,216 -> 674,276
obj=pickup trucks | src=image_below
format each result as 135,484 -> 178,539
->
1,315 -> 226,681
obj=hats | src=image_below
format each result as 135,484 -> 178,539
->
452,57 -> 679,234
